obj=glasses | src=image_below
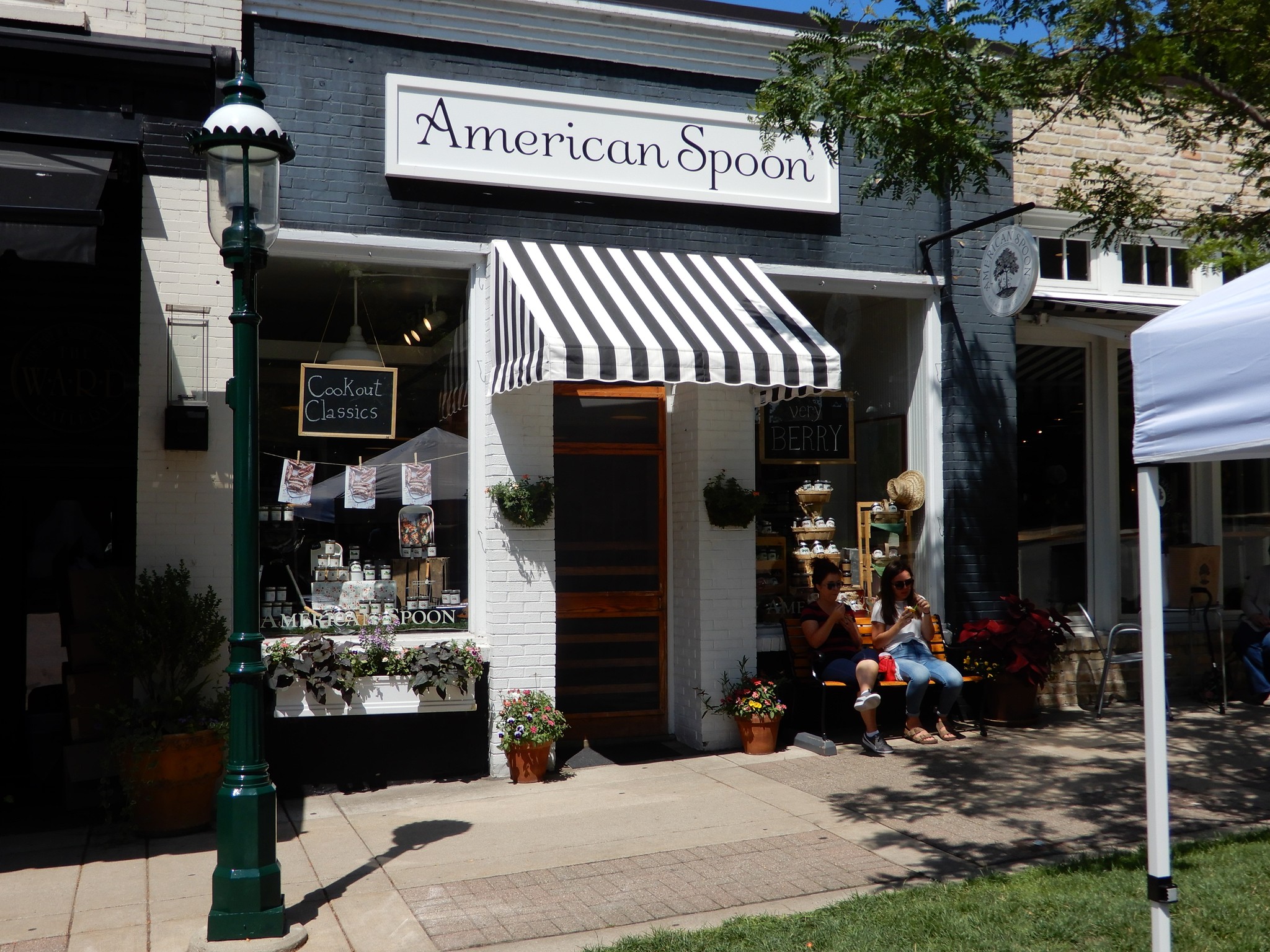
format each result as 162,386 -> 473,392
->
819,581 -> 843,590
892,578 -> 915,590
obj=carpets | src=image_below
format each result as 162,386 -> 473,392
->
557,733 -> 701,769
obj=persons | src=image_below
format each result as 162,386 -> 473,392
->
1230,542 -> 1270,707
870,561 -> 963,744
800,557 -> 894,753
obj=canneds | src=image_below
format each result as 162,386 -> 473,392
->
406,596 -> 428,609
814,517 -> 825,527
841,559 -> 852,586
873,547 -> 898,557
758,549 -> 776,560
800,542 -> 810,554
400,543 -> 436,559
314,539 -> 391,582
441,589 -> 461,605
793,517 -> 802,527
870,503 -> 882,511
756,576 -> 785,614
802,480 -> 831,491
791,559 -> 813,587
762,522 -> 772,534
825,540 -> 838,554
801,516 -> 810,527
261,587 -> 292,618
826,517 -> 835,527
889,502 -> 897,512
259,506 -> 294,521
299,599 -> 394,629
812,541 -> 824,554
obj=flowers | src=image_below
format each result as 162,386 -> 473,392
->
492,673 -> 572,754
704,467 -> 761,530
484,473 -> 557,531
263,607 -> 484,708
692,655 -> 788,720
958,593 -> 1076,690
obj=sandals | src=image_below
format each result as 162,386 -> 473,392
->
904,726 -> 938,744
935,720 -> 957,741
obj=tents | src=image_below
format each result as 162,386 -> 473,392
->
1129,263 -> 1270,951
277,424 -> 468,602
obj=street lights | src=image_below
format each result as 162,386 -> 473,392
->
184,52 -> 309,952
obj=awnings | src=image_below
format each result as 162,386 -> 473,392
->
487,236 -> 841,409
429,321 -> 467,424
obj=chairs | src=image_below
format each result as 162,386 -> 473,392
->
1076,601 -> 1175,723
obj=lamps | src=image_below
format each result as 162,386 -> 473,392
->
324,264 -> 385,368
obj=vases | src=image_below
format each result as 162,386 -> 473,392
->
268,670 -> 479,718
732,711 -> 783,755
500,735 -> 554,783
984,668 -> 1041,722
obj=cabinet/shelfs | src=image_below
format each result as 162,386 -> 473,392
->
855,500 -> 914,599
755,535 -> 787,624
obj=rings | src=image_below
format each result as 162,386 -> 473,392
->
845,623 -> 849,626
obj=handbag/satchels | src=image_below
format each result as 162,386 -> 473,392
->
877,655 -> 896,680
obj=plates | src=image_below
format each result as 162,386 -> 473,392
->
286,460 -> 314,498
350,468 -> 375,503
406,464 -> 431,499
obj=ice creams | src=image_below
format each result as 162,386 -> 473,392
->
904,606 -> 916,618
843,615 -> 853,623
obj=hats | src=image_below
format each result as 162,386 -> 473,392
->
887,469 -> 925,512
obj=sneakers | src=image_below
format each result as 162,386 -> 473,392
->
862,730 -> 893,753
854,691 -> 880,711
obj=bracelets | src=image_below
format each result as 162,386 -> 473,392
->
923,611 -> 930,614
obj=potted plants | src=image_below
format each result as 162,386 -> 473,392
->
95,559 -> 230,840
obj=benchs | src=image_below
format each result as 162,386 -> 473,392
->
780,612 -> 990,746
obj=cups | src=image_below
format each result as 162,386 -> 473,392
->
904,607 -> 916,620
844,613 -> 850,623
